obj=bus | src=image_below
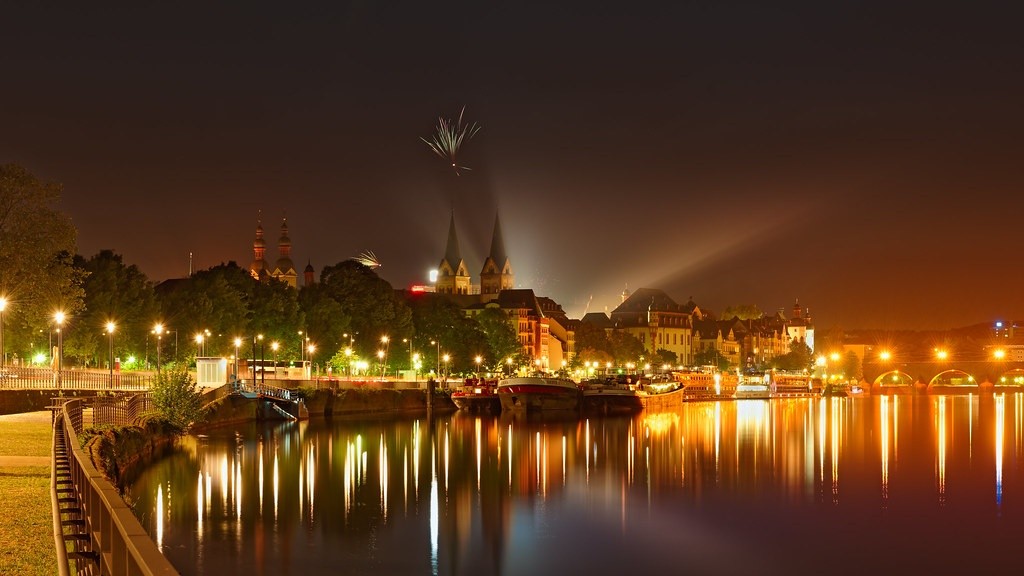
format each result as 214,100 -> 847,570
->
248,359 -> 312,381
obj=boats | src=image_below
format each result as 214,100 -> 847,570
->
832,379 -> 864,398
450,374 -> 499,410
580,379 -> 686,407
494,376 -> 579,409
733,384 -> 773,400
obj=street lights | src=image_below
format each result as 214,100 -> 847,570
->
196,334 -> 205,355
271,340 -> 279,382
233,337 -> 243,384
106,319 -> 116,391
154,324 -> 164,385
54,309 -> 67,388
